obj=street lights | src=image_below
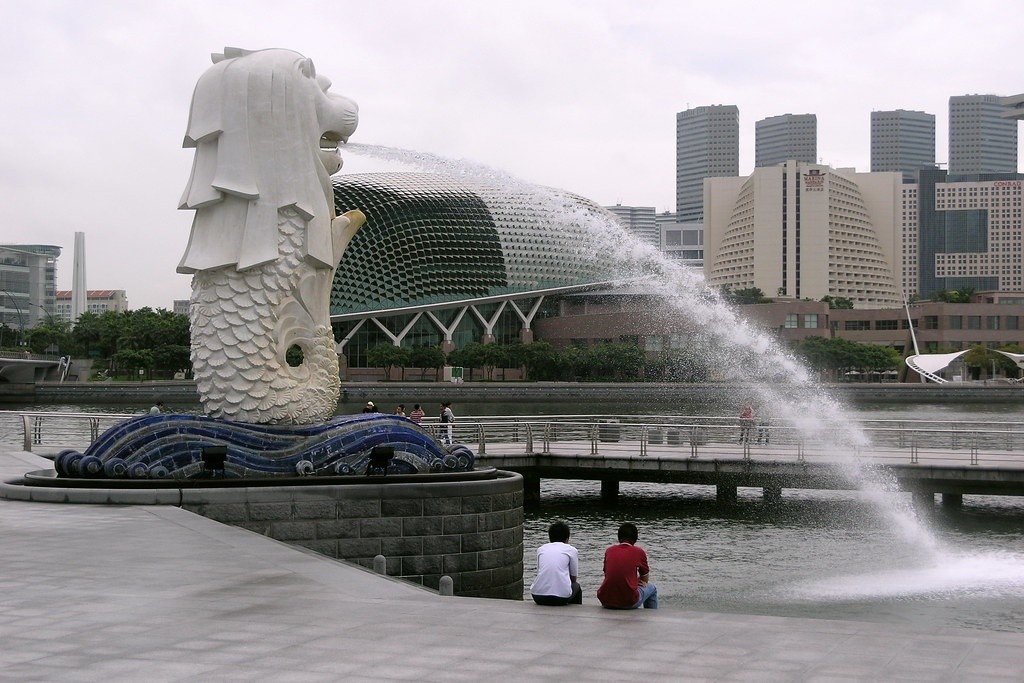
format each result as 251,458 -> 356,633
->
27,302 -> 56,325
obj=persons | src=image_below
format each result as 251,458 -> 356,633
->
395,404 -> 406,417
530,522 -> 583,606
362,401 -> 378,413
736,402 -> 756,445
150,400 -> 165,414
597,522 -> 658,610
410,404 -> 425,423
438,402 -> 455,445
24,350 -> 32,359
753,402 -> 773,445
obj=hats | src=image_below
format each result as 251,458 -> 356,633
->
367,401 -> 374,406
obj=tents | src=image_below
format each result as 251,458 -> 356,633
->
845,370 -> 899,382
